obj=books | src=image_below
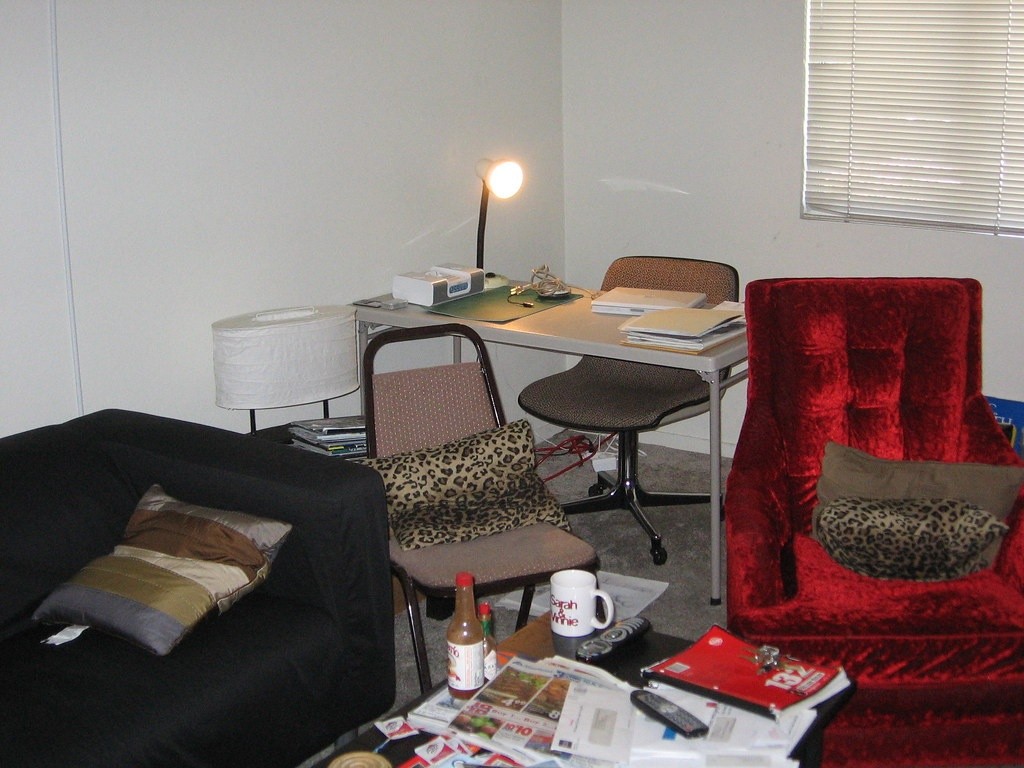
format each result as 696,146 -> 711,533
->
289,417 -> 367,463
406,653 -> 602,767
620,309 -> 746,350
642,624 -> 850,721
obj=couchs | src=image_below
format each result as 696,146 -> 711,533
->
0,409 -> 396,768
725,276 -> 1024,768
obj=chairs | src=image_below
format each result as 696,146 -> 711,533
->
363,330 -> 600,694
517,256 -> 742,566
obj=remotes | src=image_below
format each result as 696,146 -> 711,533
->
630,689 -> 710,739
575,618 -> 652,665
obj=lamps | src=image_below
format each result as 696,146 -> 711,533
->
211,303 -> 361,443
476,158 -> 524,289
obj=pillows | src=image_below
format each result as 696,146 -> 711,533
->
814,495 -> 1009,583
818,441 -> 1024,522
360,419 -> 572,553
27,482 -> 294,655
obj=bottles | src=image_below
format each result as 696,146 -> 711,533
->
477,602 -> 497,685
447,571 -> 484,699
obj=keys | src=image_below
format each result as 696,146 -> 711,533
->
744,644 -> 786,675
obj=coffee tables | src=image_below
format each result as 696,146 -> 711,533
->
307,633 -> 858,768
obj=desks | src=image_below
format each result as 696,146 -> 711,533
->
350,281 -> 748,606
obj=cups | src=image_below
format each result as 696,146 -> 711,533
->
550,569 -> 614,637
